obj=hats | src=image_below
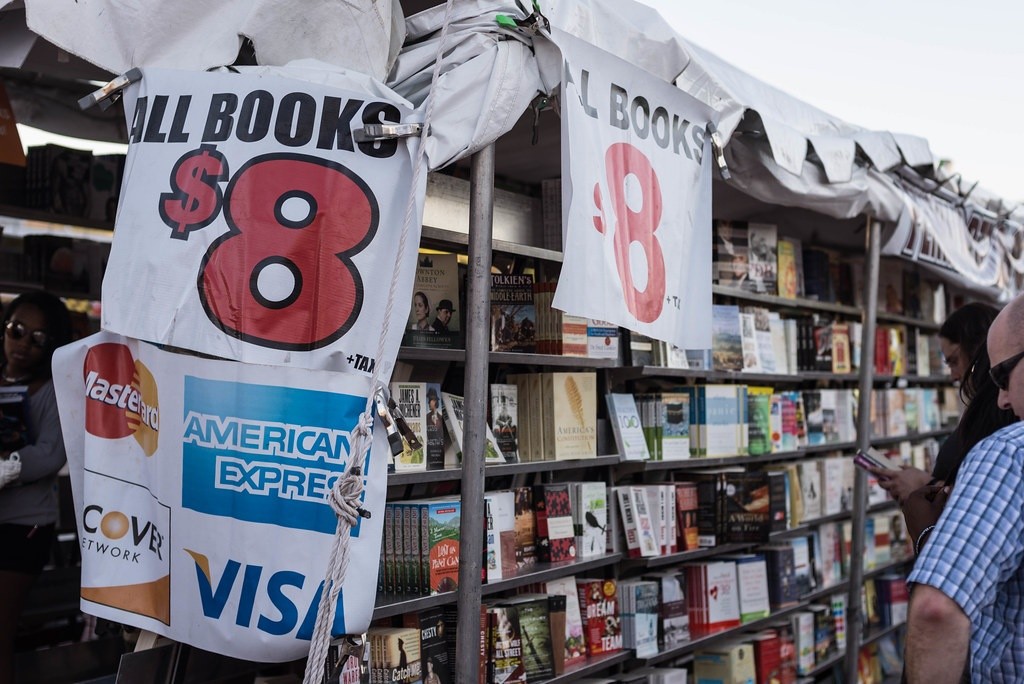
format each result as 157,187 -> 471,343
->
425,657 -> 437,664
426,387 -> 440,400
436,299 -> 456,314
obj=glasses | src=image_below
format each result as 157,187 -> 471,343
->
987,350 -> 1024,391
944,345 -> 964,369
2,319 -> 54,350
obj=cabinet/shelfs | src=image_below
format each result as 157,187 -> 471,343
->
373,166 -> 959,684
0,202 -> 117,561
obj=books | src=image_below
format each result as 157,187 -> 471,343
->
325,175 -> 968,684
2,144 -> 129,302
0,384 -> 40,488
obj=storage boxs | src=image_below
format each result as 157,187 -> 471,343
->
656,603 -> 831,684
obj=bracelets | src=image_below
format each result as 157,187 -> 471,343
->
914,525 -> 935,555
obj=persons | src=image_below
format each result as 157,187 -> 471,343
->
424,657 -> 440,684
408,292 -> 435,331
727,253 -> 757,292
496,395 -> 516,433
868,302 -> 1021,684
426,387 -> 445,469
396,638 -> 407,684
431,300 -> 457,333
0,290 -> 74,684
900,294 -> 1024,684
